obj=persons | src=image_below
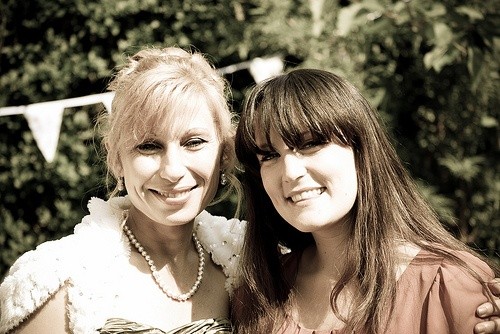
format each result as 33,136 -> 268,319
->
231,69 -> 496,334
0,44 -> 499,333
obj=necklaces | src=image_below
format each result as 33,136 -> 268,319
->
122,220 -> 204,301
